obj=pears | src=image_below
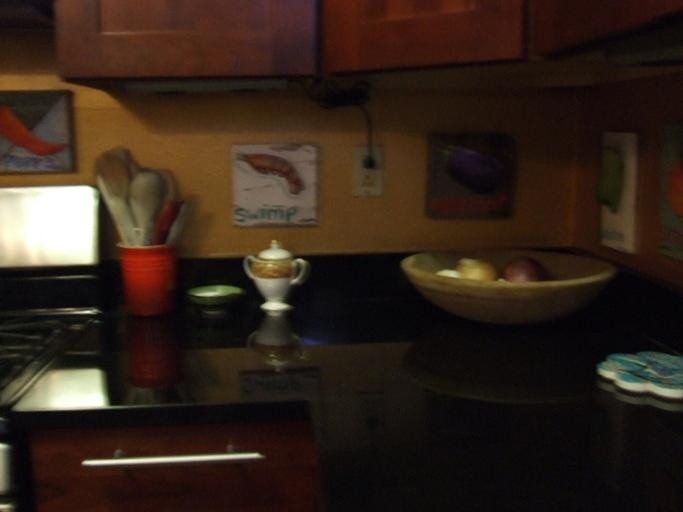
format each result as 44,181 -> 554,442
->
456,258 -> 496,280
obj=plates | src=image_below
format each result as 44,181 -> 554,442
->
185,283 -> 241,305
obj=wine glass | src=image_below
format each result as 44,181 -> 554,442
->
245,314 -> 306,374
244,237 -> 311,312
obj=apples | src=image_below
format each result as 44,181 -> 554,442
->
503,257 -> 547,282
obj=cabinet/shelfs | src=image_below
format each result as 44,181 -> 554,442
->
52,1 -> 682,94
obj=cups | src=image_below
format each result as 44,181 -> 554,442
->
115,241 -> 179,317
121,318 -> 181,387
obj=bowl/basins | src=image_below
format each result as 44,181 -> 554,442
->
398,248 -> 619,326
396,327 -> 629,406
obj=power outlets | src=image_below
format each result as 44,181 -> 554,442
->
347,144 -> 384,198
